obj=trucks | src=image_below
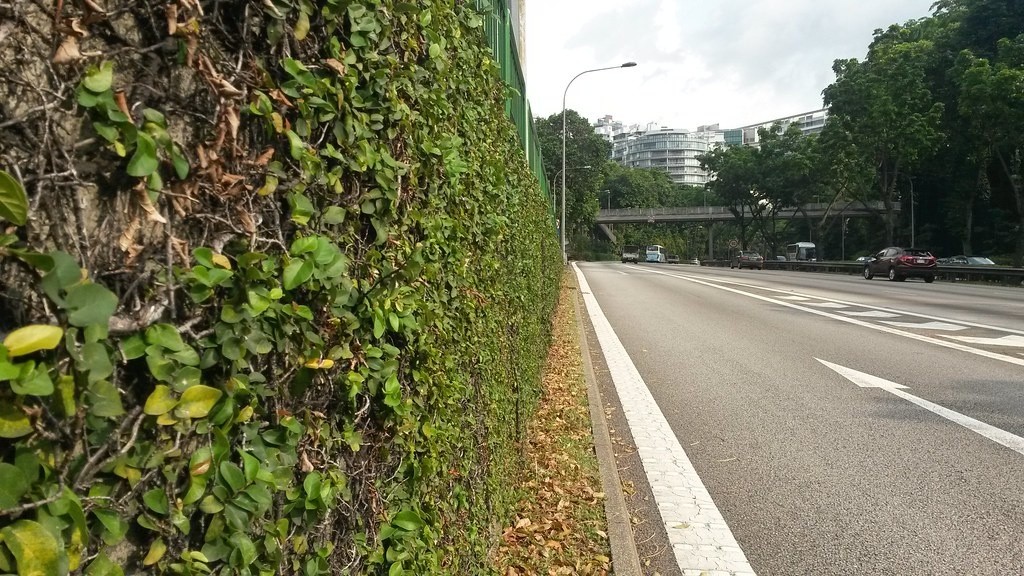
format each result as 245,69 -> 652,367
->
668,254 -> 680,264
621,245 -> 639,264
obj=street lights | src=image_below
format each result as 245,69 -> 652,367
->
553,165 -> 592,214
558,61 -> 639,266
604,189 -> 611,215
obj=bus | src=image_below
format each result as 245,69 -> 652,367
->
645,245 -> 666,263
786,242 -> 817,263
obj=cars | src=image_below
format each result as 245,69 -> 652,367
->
772,255 -> 786,262
938,255 -> 995,265
690,258 -> 700,265
863,247 -> 937,283
856,256 -> 876,263
729,250 -> 763,270
